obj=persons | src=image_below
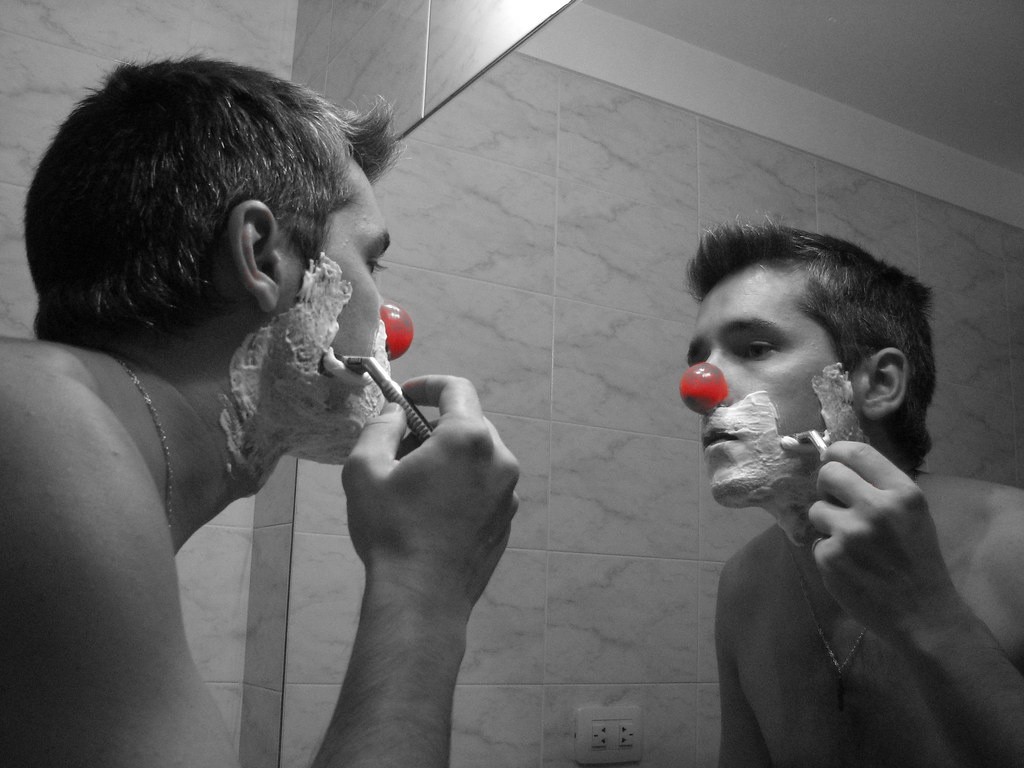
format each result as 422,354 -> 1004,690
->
0,56 -> 523,768
683,222 -> 1024,768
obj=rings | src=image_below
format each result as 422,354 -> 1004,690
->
810,537 -> 830,553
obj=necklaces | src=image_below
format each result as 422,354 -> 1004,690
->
101,351 -> 173,532
785,537 -> 867,711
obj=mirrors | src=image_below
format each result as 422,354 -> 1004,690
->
276,0 -> 1024,768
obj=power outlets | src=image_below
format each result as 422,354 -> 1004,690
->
573,705 -> 645,765
